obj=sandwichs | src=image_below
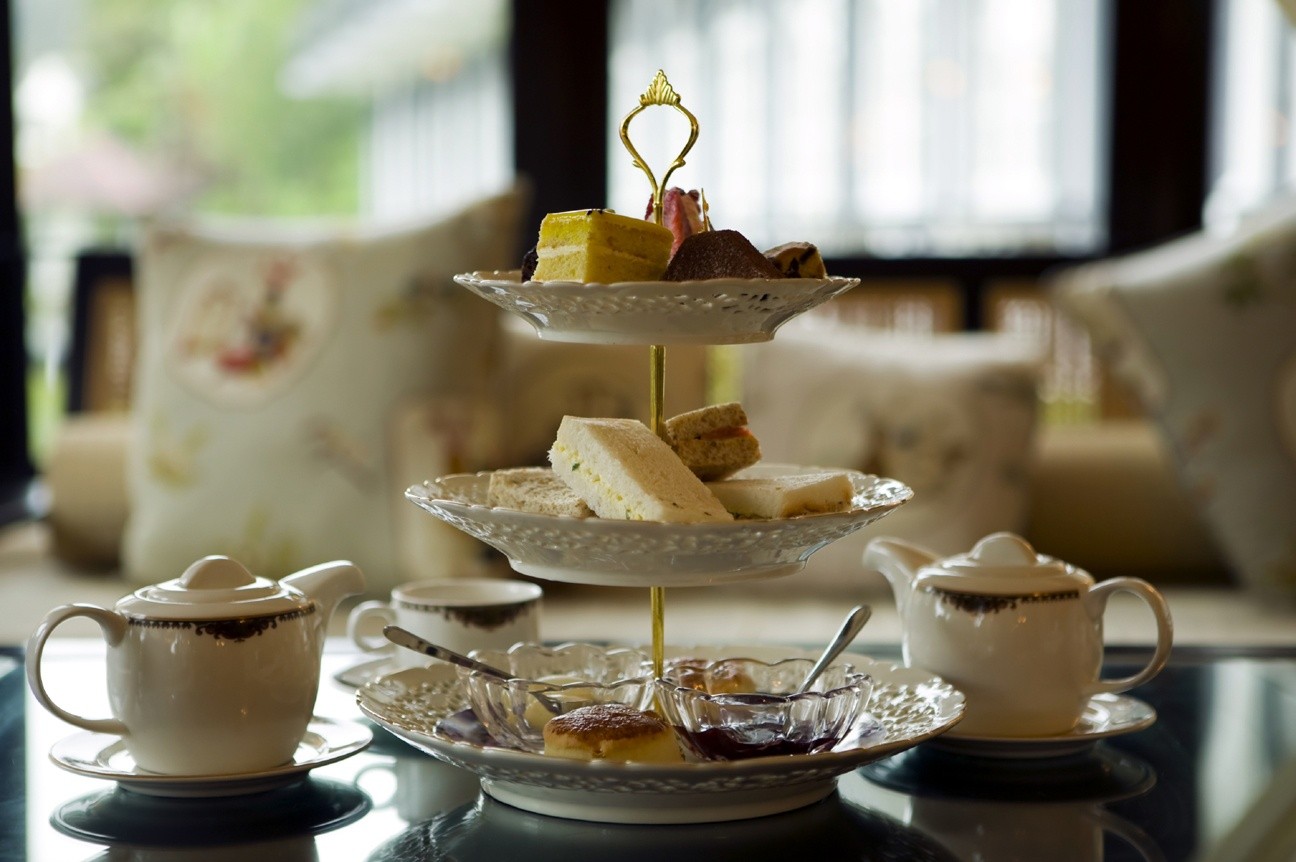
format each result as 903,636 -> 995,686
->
484,400 -> 853,530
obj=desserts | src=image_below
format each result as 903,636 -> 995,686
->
512,189 -> 827,312
542,702 -> 685,764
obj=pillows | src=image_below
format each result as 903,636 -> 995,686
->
123,182 -> 528,609
1085,194 -> 1296,617
736,317 -> 1047,607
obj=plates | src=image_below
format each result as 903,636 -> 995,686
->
331,654 -> 409,690
938,689 -> 1155,761
48,717 -> 375,797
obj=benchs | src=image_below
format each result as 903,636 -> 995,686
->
0,242 -> 1296,645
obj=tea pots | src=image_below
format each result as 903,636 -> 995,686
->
864,533 -> 1173,734
25,557 -> 374,776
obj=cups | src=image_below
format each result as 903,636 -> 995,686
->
350,577 -> 544,665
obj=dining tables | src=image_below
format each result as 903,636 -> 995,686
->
0,635 -> 1296,862
